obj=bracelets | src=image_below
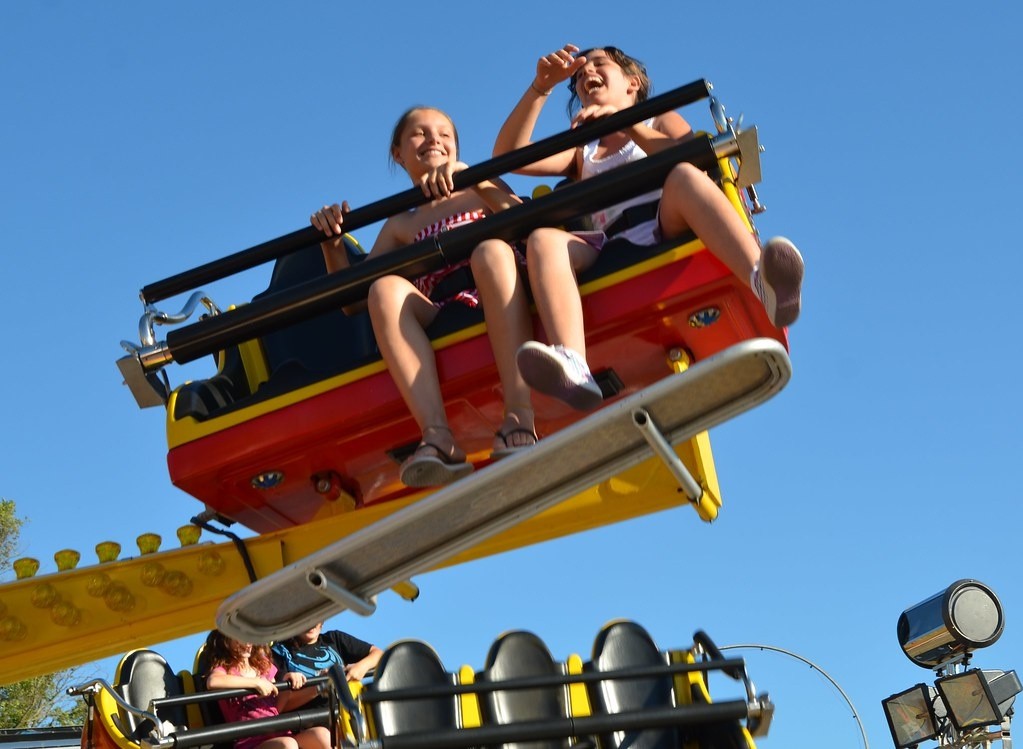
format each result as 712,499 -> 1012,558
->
532,82 -> 552,95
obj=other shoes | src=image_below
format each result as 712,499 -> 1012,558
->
761,236 -> 804,328
515,340 -> 604,412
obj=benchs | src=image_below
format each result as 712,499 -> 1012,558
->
66,75 -> 794,749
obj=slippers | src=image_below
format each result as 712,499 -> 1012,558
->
490,428 -> 538,460
400,442 -> 475,487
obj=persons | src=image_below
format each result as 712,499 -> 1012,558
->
202,620 -> 383,749
493,44 -> 805,411
311,106 -> 539,485
